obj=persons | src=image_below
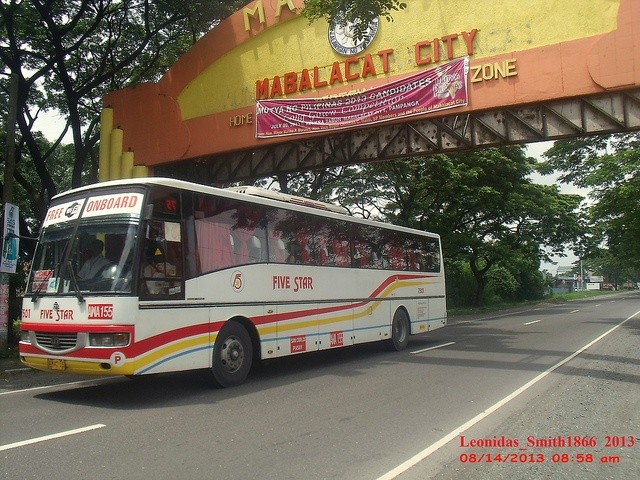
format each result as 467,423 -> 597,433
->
76,240 -> 109,282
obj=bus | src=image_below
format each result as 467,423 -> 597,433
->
18,176 -> 448,387
